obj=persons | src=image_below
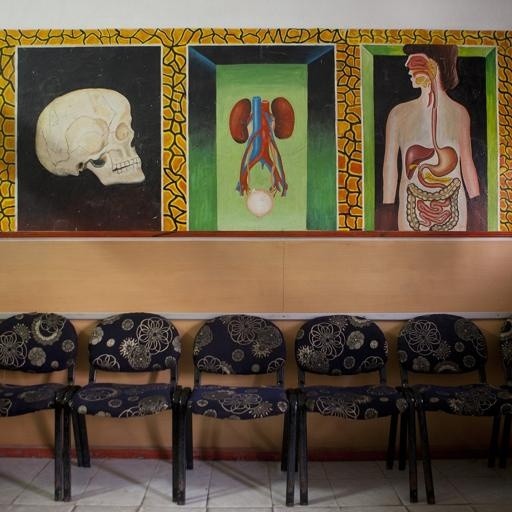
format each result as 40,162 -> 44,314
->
34,87 -> 147,186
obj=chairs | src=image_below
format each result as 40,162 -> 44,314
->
397,312 -> 511,506
297,312 -> 421,507
0,311 -> 82,504
63,311 -> 186,505
180,312 -> 298,506
